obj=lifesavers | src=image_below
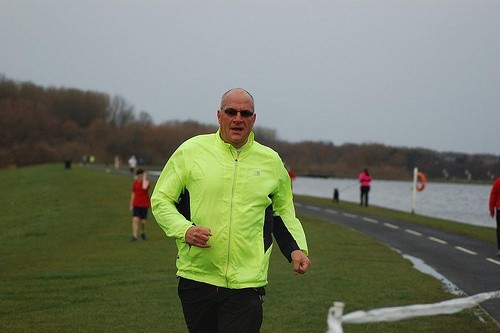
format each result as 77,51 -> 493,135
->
416,172 -> 426,192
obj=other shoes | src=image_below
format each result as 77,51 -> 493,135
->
141,233 -> 146,240
130,236 -> 137,242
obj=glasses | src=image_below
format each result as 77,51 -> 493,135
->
224,108 -> 253,118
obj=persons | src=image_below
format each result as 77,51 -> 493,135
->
359,168 -> 372,207
130,168 -> 151,242
129,155 -> 136,172
149,87 -> 311,333
489,177 -> 500,256
286,164 -> 296,180
